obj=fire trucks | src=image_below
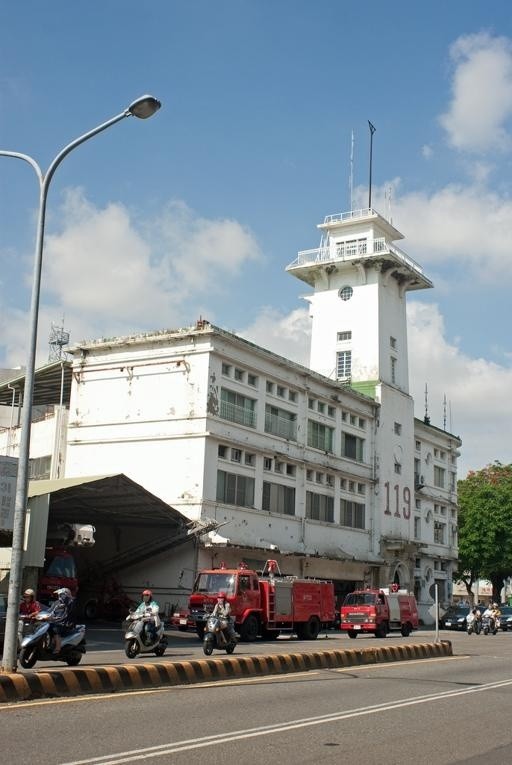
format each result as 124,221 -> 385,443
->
186,559 -> 337,642
340,582 -> 419,638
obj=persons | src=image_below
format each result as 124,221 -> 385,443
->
212,591 -> 237,643
41,588 -> 76,654
472,602 -> 501,628
19,588 -> 41,624
135,589 -> 161,645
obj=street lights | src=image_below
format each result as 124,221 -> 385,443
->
1,93 -> 164,675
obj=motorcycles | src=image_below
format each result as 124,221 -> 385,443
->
202,613 -> 240,656
465,614 -> 498,636
124,605 -> 169,659
15,600 -> 87,669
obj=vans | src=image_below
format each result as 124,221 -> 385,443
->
440,606 -> 487,631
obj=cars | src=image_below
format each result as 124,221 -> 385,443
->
170,609 -> 197,632
498,607 -> 512,631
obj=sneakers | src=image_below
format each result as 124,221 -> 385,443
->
52,649 -> 61,654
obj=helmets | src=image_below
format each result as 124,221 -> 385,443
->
217,593 -> 226,602
142,590 -> 153,601
53,588 -> 72,603
24,589 -> 35,603
